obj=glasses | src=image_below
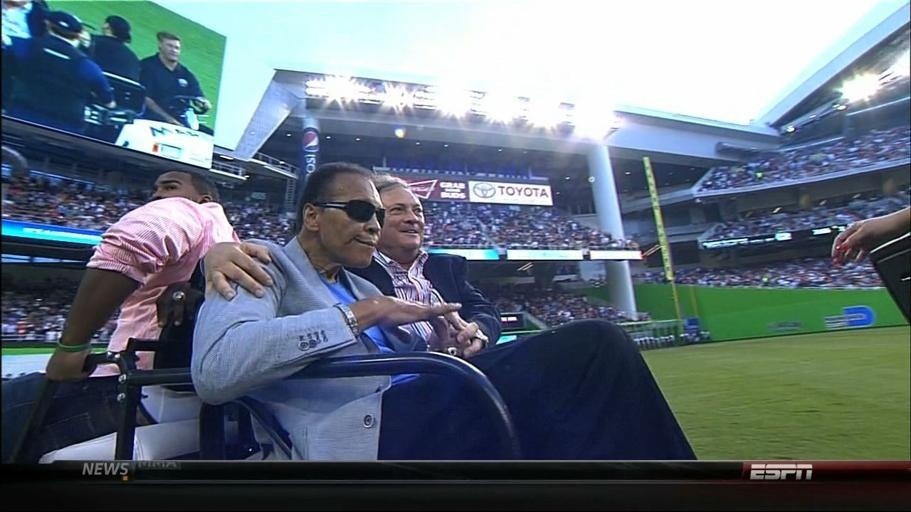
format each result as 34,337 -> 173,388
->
317,198 -> 388,227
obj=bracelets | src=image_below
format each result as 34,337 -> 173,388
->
57,336 -> 88,351
333,299 -> 361,342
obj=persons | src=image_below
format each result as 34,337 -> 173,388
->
418,202 -> 649,323
0,0 -> 49,47
649,119 -> 911,286
1,163 -> 151,341
0,10 -> 117,135
0,168 -> 243,465
190,161 -> 698,460
77,14 -> 139,83
189,172 -> 502,361
216,188 -> 299,244
139,31 -> 215,135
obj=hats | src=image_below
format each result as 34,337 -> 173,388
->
46,11 -> 83,33
105,16 -> 131,40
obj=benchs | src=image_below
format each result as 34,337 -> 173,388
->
38,339 -> 254,470
